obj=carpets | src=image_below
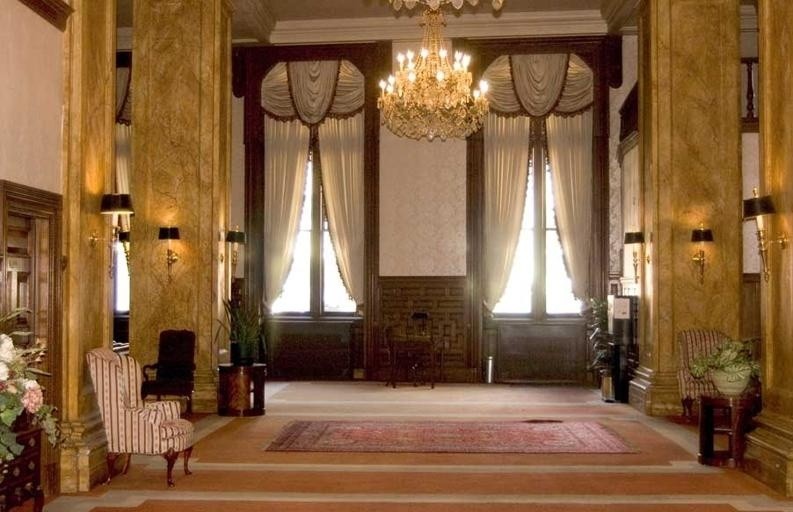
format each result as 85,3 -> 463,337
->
263,418 -> 640,456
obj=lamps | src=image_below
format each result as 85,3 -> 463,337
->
690,222 -> 712,283
89,192 -> 133,277
621,231 -> 651,285
740,187 -> 788,284
220,224 -> 245,267
374,11 -> 491,144
158,225 -> 180,278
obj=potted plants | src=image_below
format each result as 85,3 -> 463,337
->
210,296 -> 268,366
690,340 -> 760,397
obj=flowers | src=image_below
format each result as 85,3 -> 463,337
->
0,305 -> 70,461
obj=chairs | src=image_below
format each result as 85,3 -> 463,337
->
85,329 -> 197,486
674,327 -> 742,425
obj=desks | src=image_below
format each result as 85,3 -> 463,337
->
696,391 -> 759,469
215,361 -> 266,417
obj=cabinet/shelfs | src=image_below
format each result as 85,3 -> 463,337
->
0,414 -> 48,510
